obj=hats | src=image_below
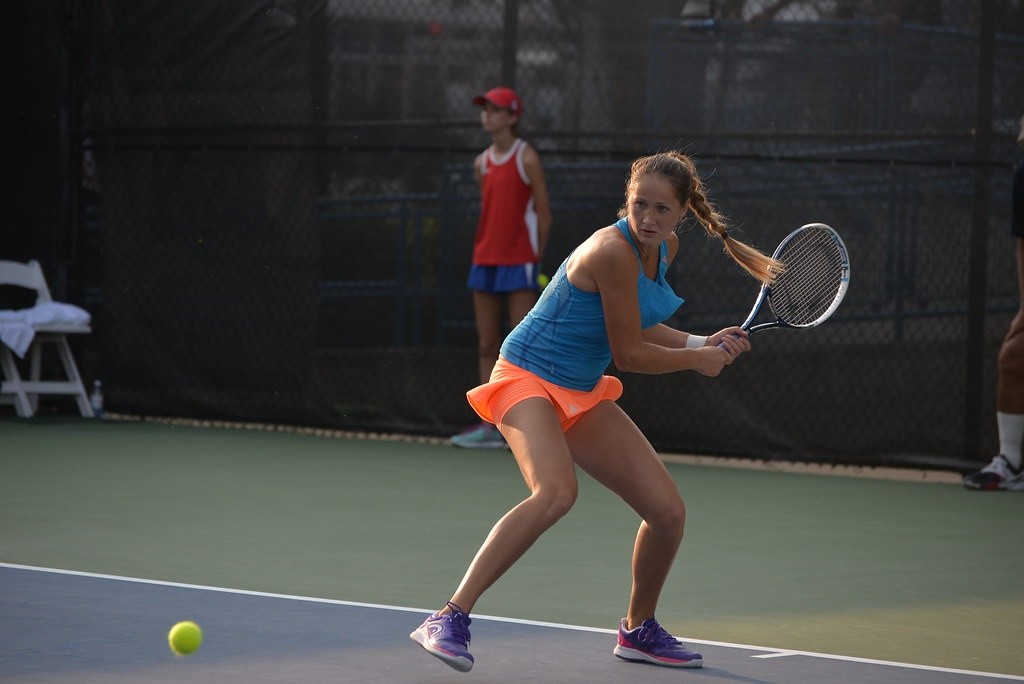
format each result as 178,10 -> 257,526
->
472,88 -> 522,117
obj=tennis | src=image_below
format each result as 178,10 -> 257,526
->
167,623 -> 204,659
536,273 -> 548,290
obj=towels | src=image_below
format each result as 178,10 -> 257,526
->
0,301 -> 91,359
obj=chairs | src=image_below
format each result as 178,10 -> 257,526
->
0,259 -> 97,420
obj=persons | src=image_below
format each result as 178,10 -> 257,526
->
957,215 -> 1023,496
410,147 -> 755,674
447,82 -> 551,449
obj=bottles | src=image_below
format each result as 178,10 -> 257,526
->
91,380 -> 103,414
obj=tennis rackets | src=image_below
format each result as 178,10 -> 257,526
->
713,226 -> 849,356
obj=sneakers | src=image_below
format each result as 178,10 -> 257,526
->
963,456 -> 1022,492
613,618 -> 703,668
450,423 -> 510,450
409,601 -> 473,673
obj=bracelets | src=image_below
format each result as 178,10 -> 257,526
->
685,334 -> 709,349
